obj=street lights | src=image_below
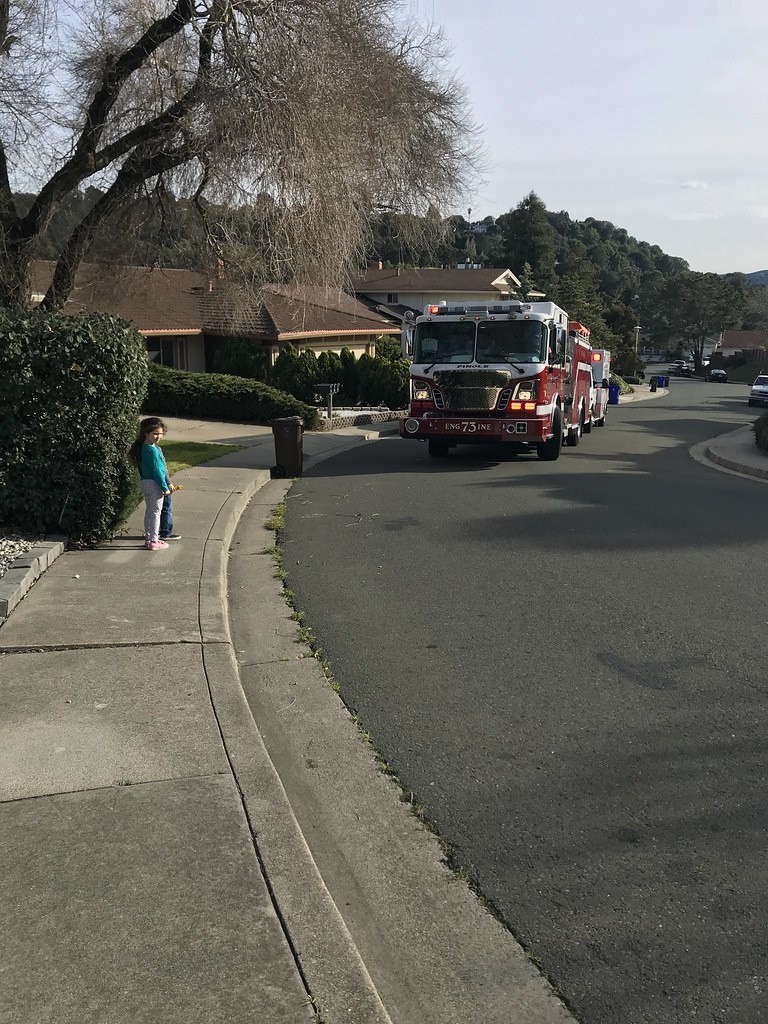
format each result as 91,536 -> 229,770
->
634,325 -> 642,377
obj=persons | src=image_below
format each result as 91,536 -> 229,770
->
129,417 -> 182,551
531,334 -> 551,361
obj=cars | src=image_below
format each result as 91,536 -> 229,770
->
748,375 -> 768,407
675,365 -> 692,378
704,369 -> 727,383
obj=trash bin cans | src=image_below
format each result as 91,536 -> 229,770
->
649,375 -> 670,392
609,385 -> 621,403
271,416 -> 305,477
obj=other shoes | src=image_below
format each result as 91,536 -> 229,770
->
159,534 -> 181,541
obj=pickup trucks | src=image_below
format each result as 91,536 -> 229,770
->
670,360 -> 686,368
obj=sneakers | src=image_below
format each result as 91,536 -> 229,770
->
145,539 -> 169,550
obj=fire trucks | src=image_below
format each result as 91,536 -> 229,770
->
400,301 -> 611,461
